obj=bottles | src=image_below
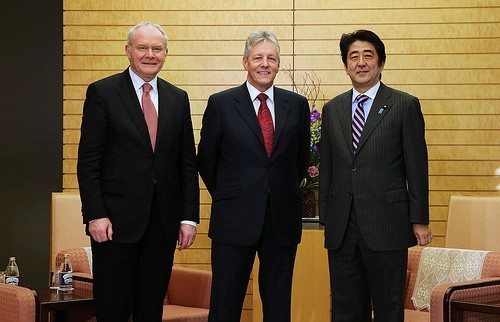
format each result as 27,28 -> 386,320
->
59,254 -> 73,294
5,257 -> 19,286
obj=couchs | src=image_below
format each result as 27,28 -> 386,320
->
56,248 -> 214,322
372,245 -> 500,322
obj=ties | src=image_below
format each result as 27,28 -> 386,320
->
256,94 -> 274,157
142,83 -> 157,152
351,94 -> 369,154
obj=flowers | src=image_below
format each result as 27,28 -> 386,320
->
300,109 -> 323,189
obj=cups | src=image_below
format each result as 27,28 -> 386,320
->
52,268 -> 60,287
0,271 -> 4,284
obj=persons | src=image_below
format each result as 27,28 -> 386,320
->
317,25 -> 433,322
195,30 -> 312,322
77,20 -> 201,322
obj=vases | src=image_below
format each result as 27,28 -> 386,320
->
300,189 -> 319,218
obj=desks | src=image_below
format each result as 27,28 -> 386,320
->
252,225 -> 333,322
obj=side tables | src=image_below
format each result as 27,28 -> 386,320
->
451,293 -> 500,322
33,289 -> 94,322
